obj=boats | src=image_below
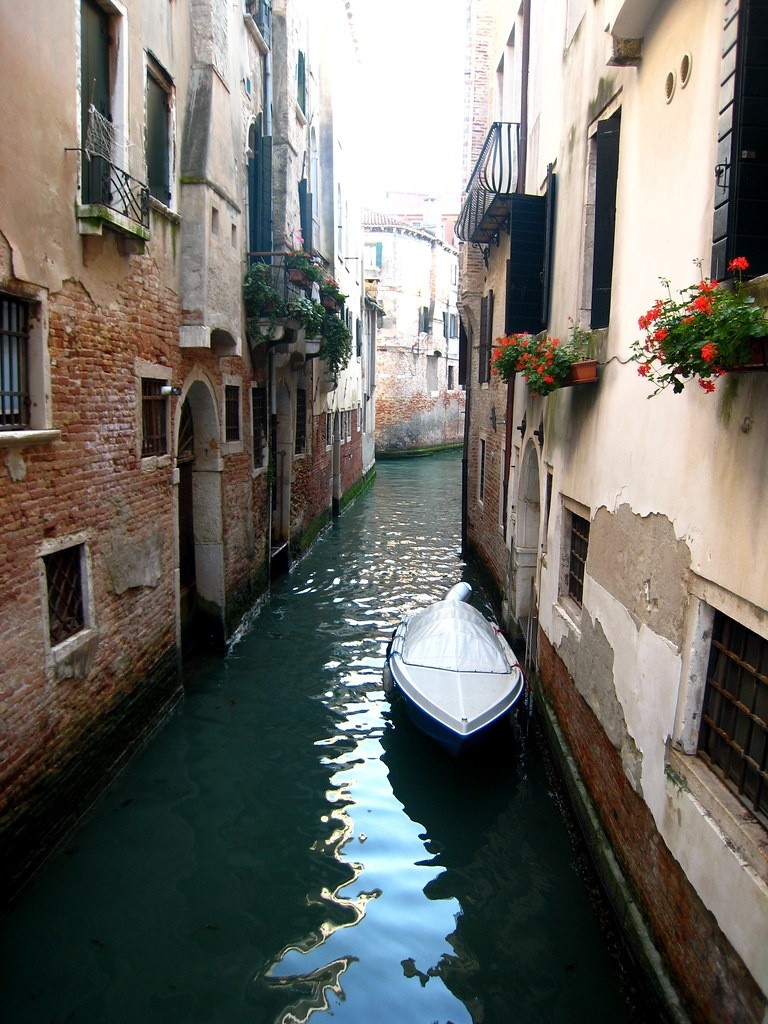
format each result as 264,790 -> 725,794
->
382,579 -> 525,757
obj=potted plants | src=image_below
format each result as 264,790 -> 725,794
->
246,268 -> 353,376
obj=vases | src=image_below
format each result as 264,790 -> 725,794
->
734,335 -> 768,371
322,296 -> 336,310
329,304 -> 339,314
567,359 -> 600,386
287,267 -> 308,287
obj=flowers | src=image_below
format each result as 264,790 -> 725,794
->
629,254 -> 750,397
275,225 -> 342,286
482,312 -> 579,399
